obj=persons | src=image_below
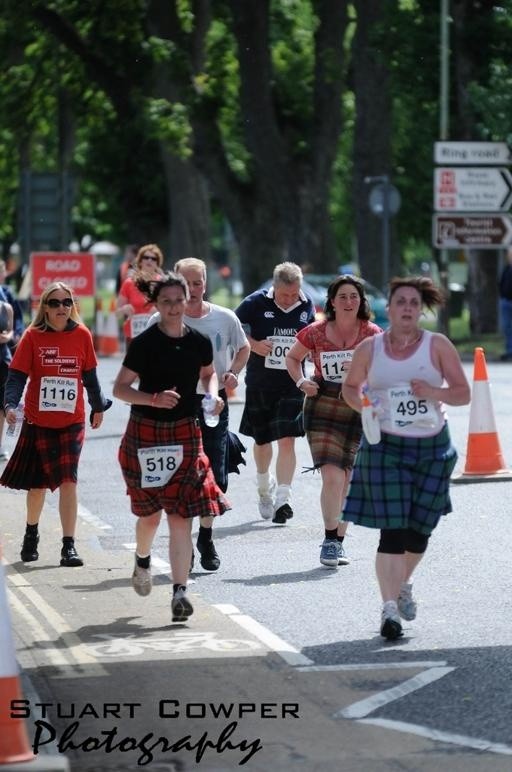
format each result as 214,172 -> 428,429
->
147,255 -> 250,575
340,275 -> 471,641
497,242 -> 510,359
1,282 -> 112,566
1,255 -> 26,463
232,260 -> 317,523
116,242 -> 164,349
113,271 -> 225,624
283,276 -> 386,567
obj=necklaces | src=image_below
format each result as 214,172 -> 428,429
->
388,328 -> 422,358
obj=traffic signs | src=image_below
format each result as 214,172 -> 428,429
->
435,170 -> 510,212
435,141 -> 510,166
431,213 -> 512,250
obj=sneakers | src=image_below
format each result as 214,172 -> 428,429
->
20,477 -> 417,639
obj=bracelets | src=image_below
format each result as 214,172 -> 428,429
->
226,370 -> 239,379
150,390 -> 160,406
293,379 -> 313,388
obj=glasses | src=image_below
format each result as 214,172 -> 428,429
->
44,299 -> 73,309
142,256 -> 159,261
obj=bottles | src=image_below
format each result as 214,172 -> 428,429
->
6,400 -> 25,440
360,398 -> 381,445
362,385 -> 385,418
201,392 -> 220,427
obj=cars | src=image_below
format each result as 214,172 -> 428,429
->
255,273 -> 390,335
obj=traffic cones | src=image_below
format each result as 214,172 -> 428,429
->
0,560 -> 38,764
92,297 -> 104,353
29,298 -> 42,322
75,298 -> 84,322
98,297 -> 121,359
452,347 -> 510,484
